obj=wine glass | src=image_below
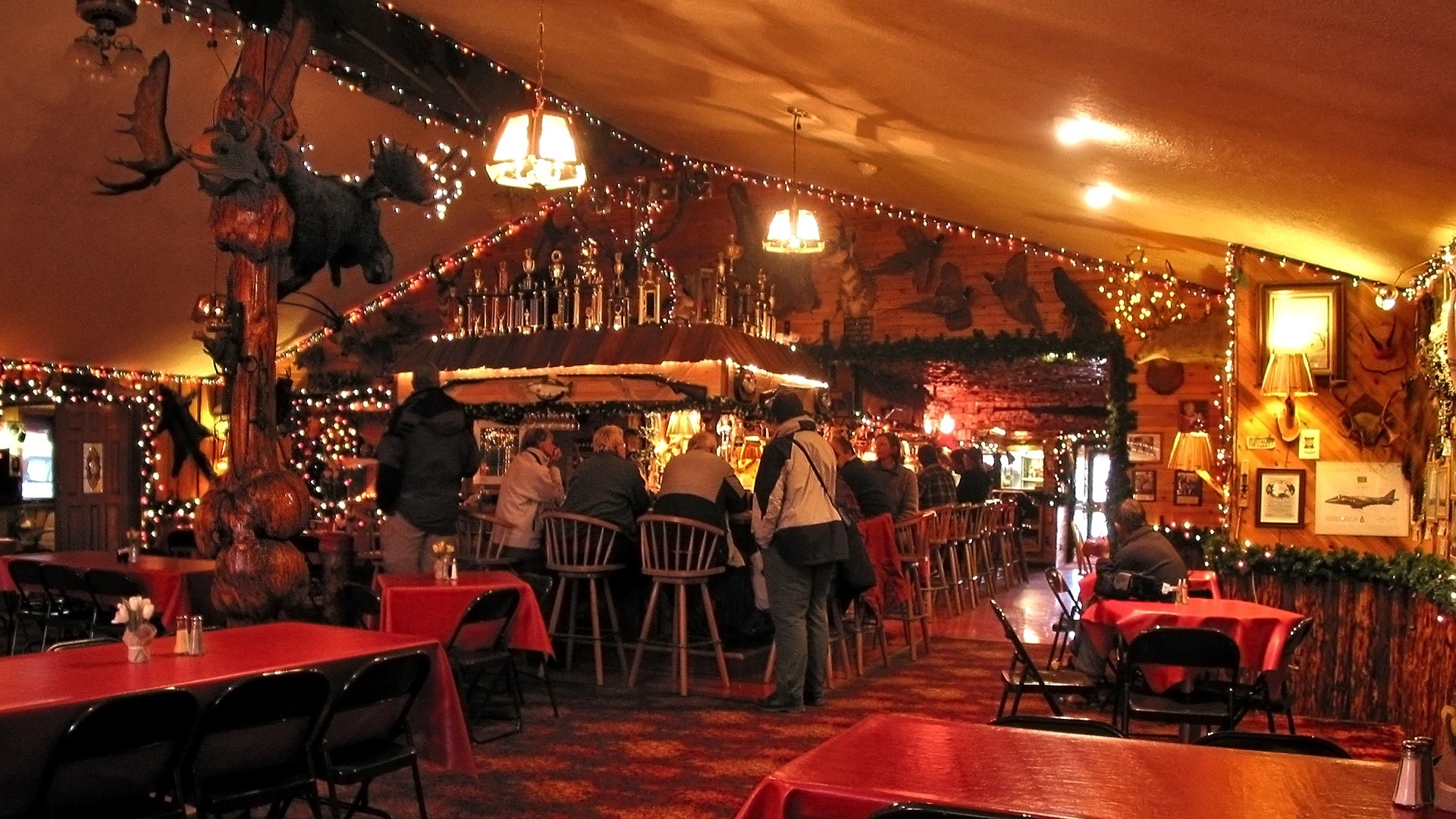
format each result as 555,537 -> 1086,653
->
546,411 -> 579,431
520,412 -> 546,430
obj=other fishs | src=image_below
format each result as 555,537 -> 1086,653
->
528,381 -> 572,401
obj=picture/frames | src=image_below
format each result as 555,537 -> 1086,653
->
1173,470 -> 1203,507
1256,468 -> 1305,528
1132,470 -> 1156,502
1126,432 -> 1163,466
1256,279 -> 1347,388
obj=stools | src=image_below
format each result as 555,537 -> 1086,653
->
459,499 -> 1020,694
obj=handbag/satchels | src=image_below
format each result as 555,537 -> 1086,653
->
836,522 -> 876,600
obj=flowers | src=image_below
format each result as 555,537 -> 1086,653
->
110,595 -> 155,628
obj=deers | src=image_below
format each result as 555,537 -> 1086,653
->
566,168 -> 684,318
1122,259 -> 1233,363
819,211 -> 876,316
428,254 -> 491,326
277,134 -> 470,303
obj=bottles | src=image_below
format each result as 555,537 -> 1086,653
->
174,614 -> 189,654
482,428 -> 518,466
1182,578 -> 1189,604
1392,740 -> 1426,809
129,540 -> 138,563
189,616 -> 205,655
450,558 -> 458,580
443,557 -> 450,581
1414,736 -> 1434,805
1174,579 -> 1183,606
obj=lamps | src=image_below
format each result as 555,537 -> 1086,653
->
485,0 -> 587,190
759,106 -> 825,254
1261,349 -> 1315,443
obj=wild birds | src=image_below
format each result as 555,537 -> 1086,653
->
879,262 -> 976,331
1051,267 -> 1107,328
862,225 -> 947,294
979,252 -> 1045,333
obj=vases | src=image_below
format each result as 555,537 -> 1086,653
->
122,623 -> 157,663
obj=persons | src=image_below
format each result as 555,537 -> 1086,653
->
751,390 -> 850,713
374,358 -> 480,572
1068,498 -> 1187,679
490,424 -> 991,649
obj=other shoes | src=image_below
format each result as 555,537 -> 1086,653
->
804,690 -> 826,706
1058,694 -> 1101,713
755,692 -> 805,714
1052,657 -> 1073,671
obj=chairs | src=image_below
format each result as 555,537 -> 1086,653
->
1114,625 -> 1249,740
1229,616 -> 1313,734
48,651 -> 430,819
447,588 -> 523,744
1044,567 -> 1079,670
7,559 -> 168,655
990,600 -> 1093,716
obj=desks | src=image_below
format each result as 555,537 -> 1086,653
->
0,551 -> 217,634
1079,572 -> 1222,602
364,573 -> 560,718
0,623 -> 480,819
1081,599 -> 1304,693
732,714 -> 1456,819
